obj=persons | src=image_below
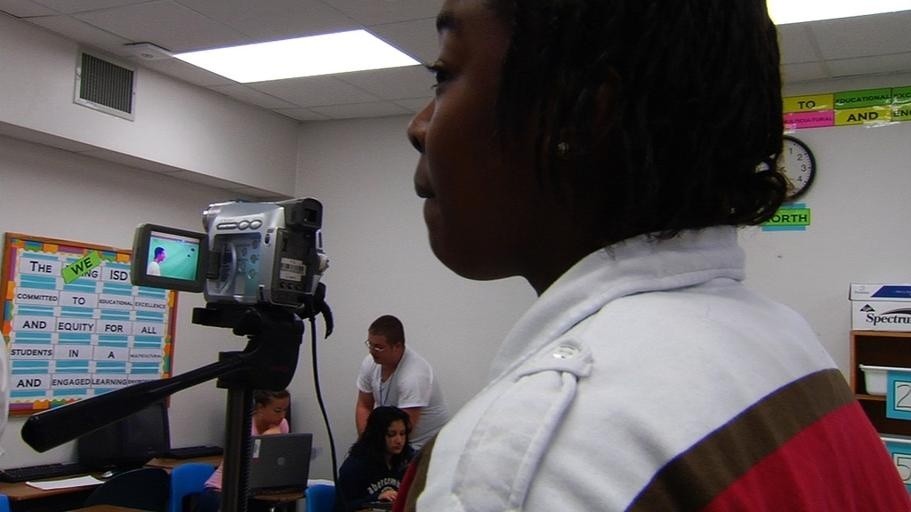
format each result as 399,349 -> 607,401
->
356,314 -> 450,511
146,247 -> 166,277
197,388 -> 290,511
336,405 -> 417,512
405,0 -> 911,512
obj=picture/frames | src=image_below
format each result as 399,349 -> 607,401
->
0,231 -> 179,419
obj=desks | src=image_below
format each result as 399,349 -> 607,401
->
253,489 -> 302,511
2,450 -> 226,503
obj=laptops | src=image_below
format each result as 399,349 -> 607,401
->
248,433 -> 313,490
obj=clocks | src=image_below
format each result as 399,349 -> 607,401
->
759,130 -> 816,203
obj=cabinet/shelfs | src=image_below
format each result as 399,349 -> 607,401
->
849,330 -> 911,401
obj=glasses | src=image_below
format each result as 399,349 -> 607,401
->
365,339 -> 390,352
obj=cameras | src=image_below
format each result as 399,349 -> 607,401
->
127,196 -> 332,316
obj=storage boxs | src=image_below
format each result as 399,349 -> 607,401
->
859,362 -> 911,397
850,284 -> 911,330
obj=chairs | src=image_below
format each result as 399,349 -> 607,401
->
170,462 -> 215,511
88,469 -> 169,504
304,485 -> 341,511
